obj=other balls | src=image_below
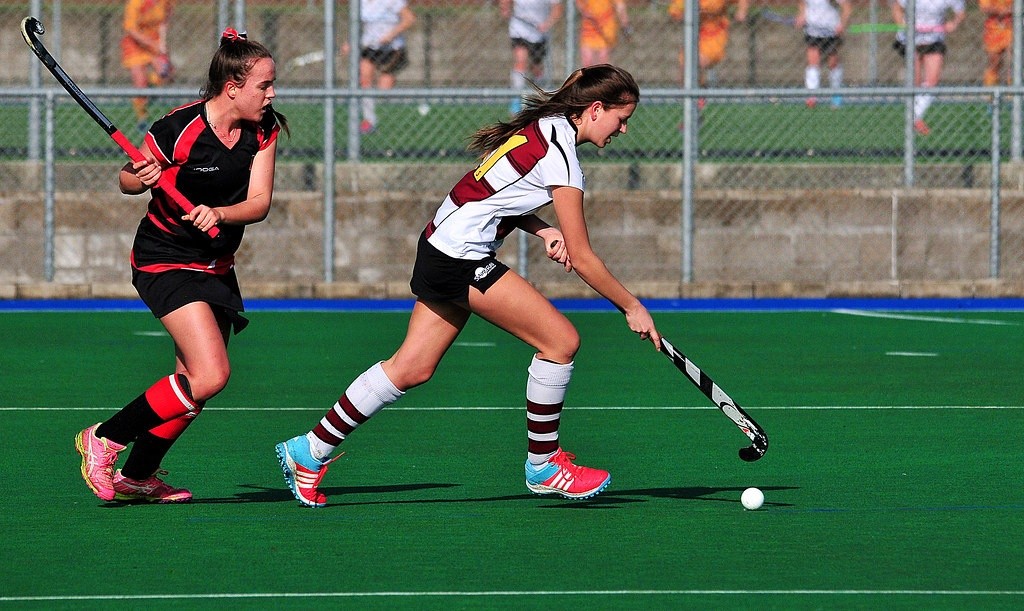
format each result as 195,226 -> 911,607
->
740,486 -> 766,511
418,104 -> 431,116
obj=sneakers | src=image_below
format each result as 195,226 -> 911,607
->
110,466 -> 192,505
274,432 -> 345,507
525,445 -> 611,500
75,423 -> 127,500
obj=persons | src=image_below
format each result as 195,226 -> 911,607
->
274,62 -> 664,509
795,0 -> 853,110
668,0 -> 750,134
339,1 -> 415,135
120,1 -> 172,132
891,0 -> 968,134
979,1 -> 1014,104
497,1 -> 564,115
571,1 -> 628,70
74,28 -> 290,502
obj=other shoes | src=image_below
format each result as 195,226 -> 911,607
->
695,99 -> 705,108
136,119 -> 153,131
363,119 -> 372,133
915,119 -> 928,134
802,98 -> 814,105
828,98 -> 843,104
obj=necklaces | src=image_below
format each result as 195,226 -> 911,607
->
206,101 -> 236,142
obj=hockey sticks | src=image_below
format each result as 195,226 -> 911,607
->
549,239 -> 769,462
19,14 -> 222,241
762,7 -> 832,32
293,40 -> 379,67
849,22 -> 944,35
482,2 -> 538,28
